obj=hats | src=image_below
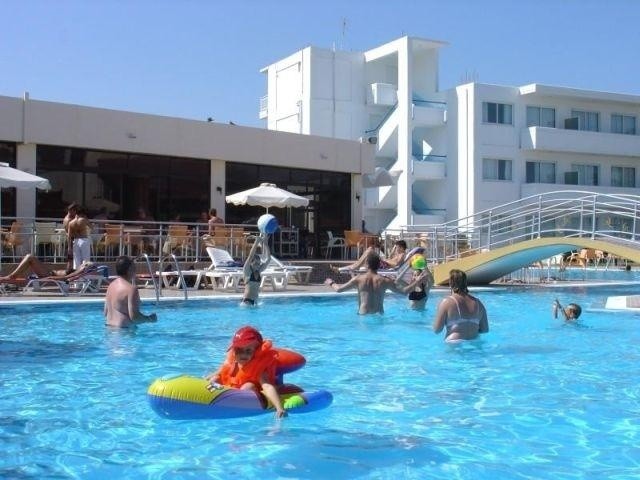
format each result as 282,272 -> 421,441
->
225,328 -> 262,352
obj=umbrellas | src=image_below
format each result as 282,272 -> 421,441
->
225,181 -> 310,214
0,161 -> 52,195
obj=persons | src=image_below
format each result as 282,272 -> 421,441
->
103,255 -> 159,331
63,201 -> 76,272
194,211 -> 209,233
0,253 -> 92,283
67,207 -> 94,270
241,234 -> 270,311
554,297 -> 581,322
136,208 -> 157,253
167,213 -> 190,239
199,208 -> 222,257
394,260 -> 433,312
329,239 -> 407,272
207,324 -> 289,421
324,251 -> 431,318
434,268 -> 488,343
1,219 -> 21,249
91,204 -> 109,224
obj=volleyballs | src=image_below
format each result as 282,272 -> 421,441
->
258,214 -> 277,234
411,254 -> 425,269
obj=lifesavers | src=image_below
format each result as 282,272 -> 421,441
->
147,347 -> 333,421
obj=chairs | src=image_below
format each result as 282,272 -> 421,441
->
324,228 -> 427,289
1,215 -> 316,298
